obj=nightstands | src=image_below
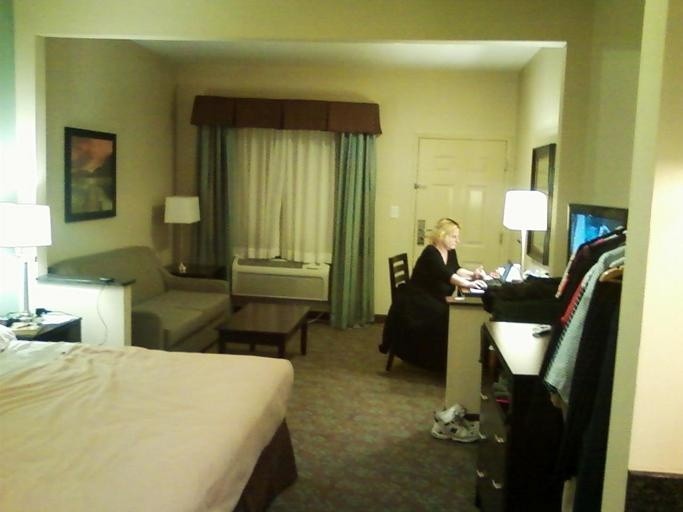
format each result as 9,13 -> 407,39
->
16,311 -> 82,342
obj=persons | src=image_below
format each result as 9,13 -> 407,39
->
378,217 -> 490,376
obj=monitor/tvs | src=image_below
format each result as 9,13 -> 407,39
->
567,204 -> 628,265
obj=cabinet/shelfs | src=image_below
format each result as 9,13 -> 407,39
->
475,334 -> 561,511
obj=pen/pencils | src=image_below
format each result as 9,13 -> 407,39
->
17,323 -> 32,329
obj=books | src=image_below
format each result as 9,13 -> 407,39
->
8,320 -> 42,336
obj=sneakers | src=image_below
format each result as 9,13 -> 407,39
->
430,420 -> 479,443
431,410 -> 480,431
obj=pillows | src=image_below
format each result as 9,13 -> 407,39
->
0,323 -> 15,353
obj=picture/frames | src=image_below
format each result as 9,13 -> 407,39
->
63,125 -> 117,224
526,143 -> 555,266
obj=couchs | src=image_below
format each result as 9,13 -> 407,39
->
48,245 -> 232,352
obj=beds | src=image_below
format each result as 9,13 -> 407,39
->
1,341 -> 294,511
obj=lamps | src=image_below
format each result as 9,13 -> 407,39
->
502,189 -> 549,273
164,194 -> 202,273
0,203 -> 52,318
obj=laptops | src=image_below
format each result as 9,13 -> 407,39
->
484,260 -> 513,287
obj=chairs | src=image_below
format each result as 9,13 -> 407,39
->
385,253 -> 410,372
